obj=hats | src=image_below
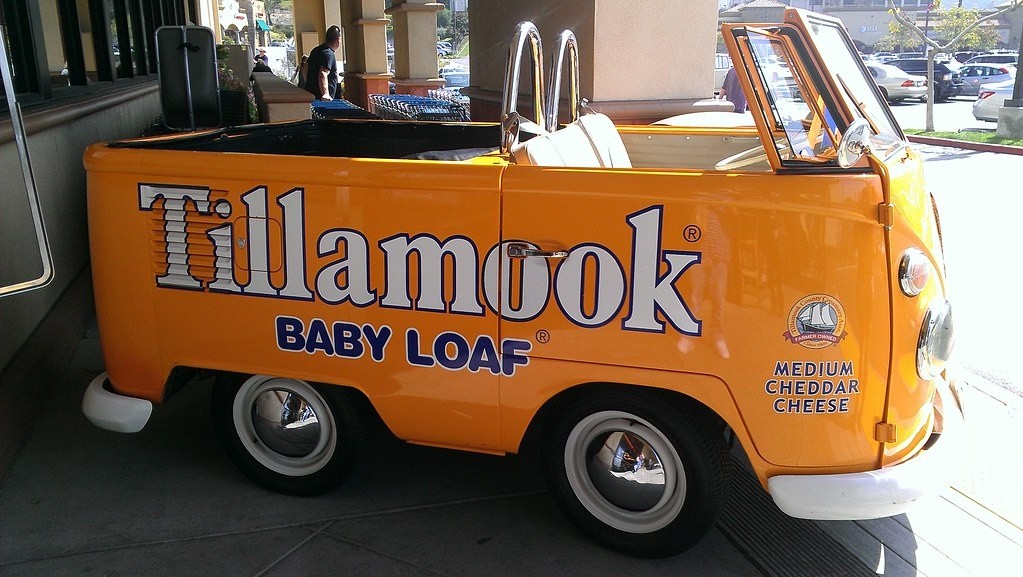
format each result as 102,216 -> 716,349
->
259,48 -> 266,53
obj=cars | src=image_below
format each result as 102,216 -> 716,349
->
74,9 -> 1021,543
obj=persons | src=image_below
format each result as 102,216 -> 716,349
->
253,47 -> 268,66
249,60 -> 273,82
301,25 -> 342,100
298,54 -> 310,91
718,65 -> 748,114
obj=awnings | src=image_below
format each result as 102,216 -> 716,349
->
256,19 -> 271,32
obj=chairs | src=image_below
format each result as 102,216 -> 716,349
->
511,111 -> 633,171
